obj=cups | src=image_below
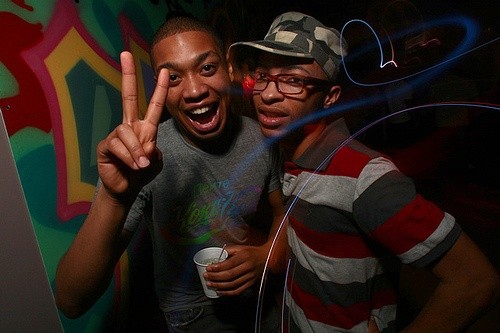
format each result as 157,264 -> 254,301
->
193,247 -> 229,298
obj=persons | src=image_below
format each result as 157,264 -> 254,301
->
225,8 -> 500,332
45,10 -> 298,332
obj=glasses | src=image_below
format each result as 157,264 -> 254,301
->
242,65 -> 336,95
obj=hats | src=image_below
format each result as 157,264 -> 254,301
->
227,12 -> 348,81
